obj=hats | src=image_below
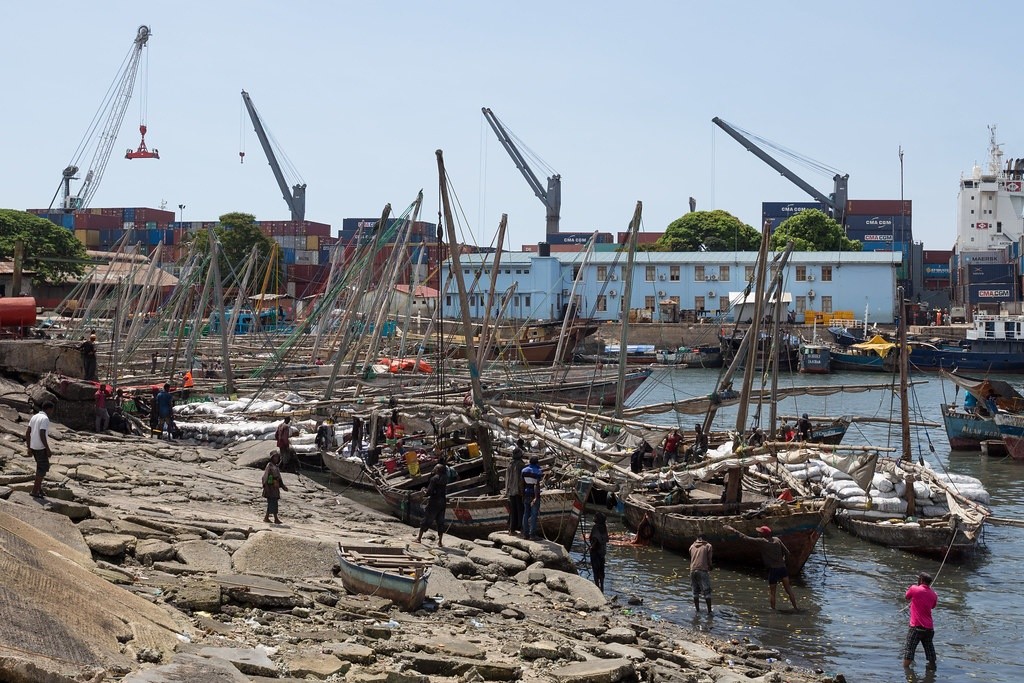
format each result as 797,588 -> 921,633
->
270,450 -> 280,460
511,448 -> 525,459
756,526 -> 771,535
915,571 -> 934,583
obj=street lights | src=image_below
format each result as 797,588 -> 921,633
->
178,204 -> 186,277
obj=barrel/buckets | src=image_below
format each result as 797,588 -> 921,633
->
468,442 -> 480,458
385,459 -> 397,475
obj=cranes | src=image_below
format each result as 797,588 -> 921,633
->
477,106 -> 561,243
711,117 -> 849,236
45,24 -> 160,236
239,88 -> 308,222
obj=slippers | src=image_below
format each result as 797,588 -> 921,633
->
31,493 -> 44,497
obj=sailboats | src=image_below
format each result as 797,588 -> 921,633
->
32,148 -> 990,577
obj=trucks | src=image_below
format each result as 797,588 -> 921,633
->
951,308 -> 966,324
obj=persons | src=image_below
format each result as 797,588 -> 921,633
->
496,309 -> 499,317
794,413 -> 813,443
788,310 -> 797,320
262,450 -> 288,524
315,358 -> 323,365
662,430 -> 683,467
26,401 -> 53,498
631,444 -> 645,474
903,572 -> 937,668
182,370 -> 194,389
113,389 -> 124,414
505,448 -> 542,541
75,334 -> 97,377
685,424 -> 708,461
94,384 -> 110,433
723,524 -> 798,609
588,512 -> 609,592
746,426 -> 767,447
150,383 -> 173,440
784,424 -> 794,448
412,463 -> 447,547
689,534 -> 714,613
927,310 -> 948,326
964,390 -> 977,414
275,416 -> 291,471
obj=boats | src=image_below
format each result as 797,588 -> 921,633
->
718,293 -> 1024,375
940,359 -> 1024,460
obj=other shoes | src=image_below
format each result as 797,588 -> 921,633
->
523,534 -> 530,539
509,531 -> 517,535
530,536 -> 542,541
